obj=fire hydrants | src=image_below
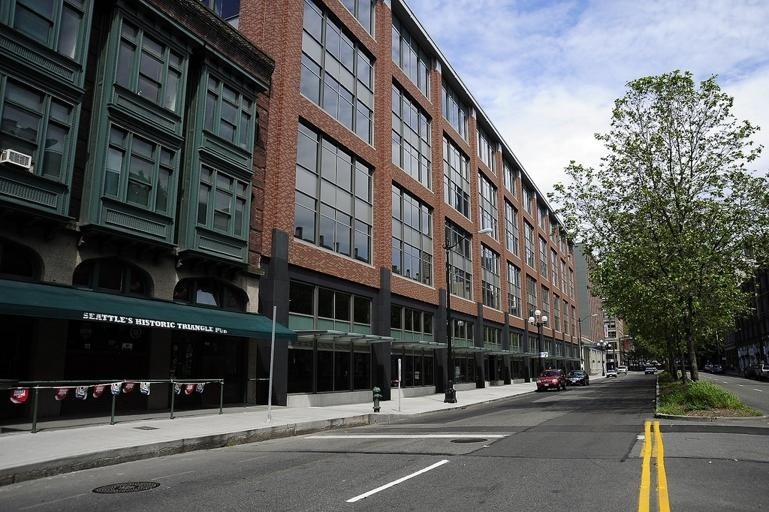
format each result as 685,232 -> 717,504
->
372,386 -> 382,412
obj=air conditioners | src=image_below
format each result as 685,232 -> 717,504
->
0,149 -> 32,169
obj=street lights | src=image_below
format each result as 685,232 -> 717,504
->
443,228 -> 493,403
579,313 -> 599,372
528,310 -> 547,372
618,333 -> 629,365
597,339 -> 610,375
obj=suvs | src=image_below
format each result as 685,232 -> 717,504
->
537,369 -> 566,391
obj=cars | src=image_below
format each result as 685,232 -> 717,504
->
645,366 -> 657,375
705,364 -> 722,372
567,370 -> 589,385
607,370 -> 617,378
618,366 -> 628,375
